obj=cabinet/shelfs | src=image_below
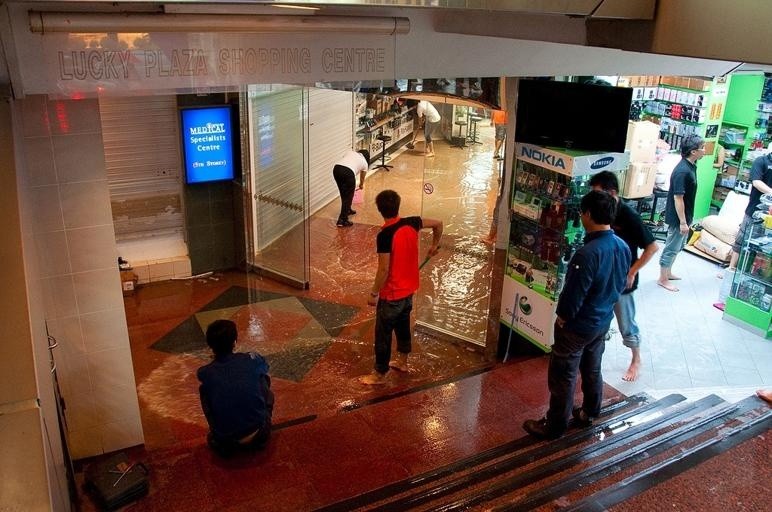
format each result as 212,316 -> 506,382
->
713,121 -> 752,208
357,104 -> 418,161
623,75 -> 710,156
721,216 -> 772,341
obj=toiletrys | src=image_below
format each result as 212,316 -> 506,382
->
719,267 -> 736,301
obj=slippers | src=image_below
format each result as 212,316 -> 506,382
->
493,155 -> 500,158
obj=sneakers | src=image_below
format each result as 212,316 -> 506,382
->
572,405 -> 592,427
347,209 -> 356,215
336,218 -> 353,227
522,416 -> 562,438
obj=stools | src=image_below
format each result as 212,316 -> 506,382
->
372,137 -> 393,172
650,186 -> 668,231
456,110 -> 483,146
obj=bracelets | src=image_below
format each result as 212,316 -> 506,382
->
679,222 -> 687,225
370,291 -> 378,297
557,317 -> 565,326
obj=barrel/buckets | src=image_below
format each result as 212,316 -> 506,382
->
352,189 -> 364,204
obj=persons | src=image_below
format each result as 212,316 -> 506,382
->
332,149 -> 370,227
756,389 -> 772,403
716,141 -> 772,279
656,135 -> 705,292
197,320 -> 274,459
521,190 -> 631,440
406,99 -> 441,157
490,108 -> 509,158
712,144 -> 726,199
358,190 -> 443,385
590,170 -> 659,383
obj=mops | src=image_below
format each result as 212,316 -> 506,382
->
406,125 -> 419,148
